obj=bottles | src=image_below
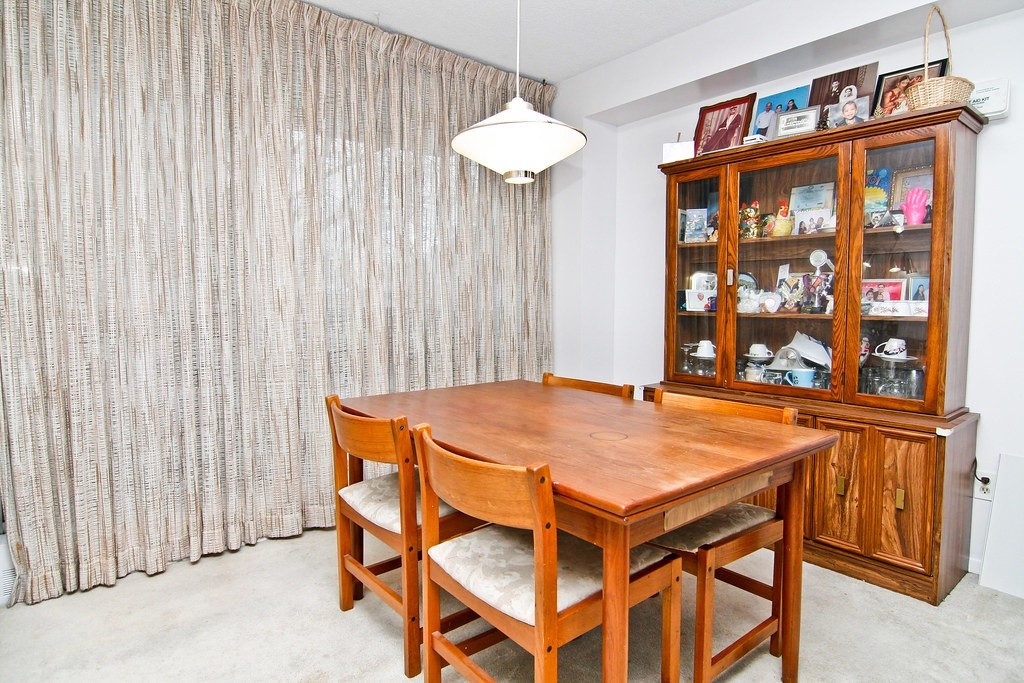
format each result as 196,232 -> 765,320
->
682,332 -> 926,404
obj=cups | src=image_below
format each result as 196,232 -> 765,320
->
874,337 -> 908,359
695,340 -> 716,356
783,367 -> 816,387
748,343 -> 773,357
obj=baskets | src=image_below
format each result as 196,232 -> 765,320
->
905,5 -> 975,112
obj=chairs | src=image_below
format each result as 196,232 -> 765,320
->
325,372 -> 799,683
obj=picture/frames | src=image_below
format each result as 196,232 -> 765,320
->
889,164 -> 934,225
870,58 -> 949,120
795,209 -> 831,235
676,209 -> 687,244
908,276 -> 929,301
693,92 -> 756,158
773,104 -> 820,139
861,278 -> 907,305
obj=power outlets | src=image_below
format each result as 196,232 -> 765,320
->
974,472 -> 995,502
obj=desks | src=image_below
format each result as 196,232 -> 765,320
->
335,380 -> 839,683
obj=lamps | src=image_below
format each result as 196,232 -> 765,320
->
450,0 -> 588,185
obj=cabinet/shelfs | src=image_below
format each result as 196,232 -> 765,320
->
643,102 -> 990,607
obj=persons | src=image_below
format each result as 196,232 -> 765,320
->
703,106 -> 741,152
862,284 -> 884,302
825,80 -> 842,104
913,285 -> 925,300
839,101 -> 863,126
786,99 -> 798,111
755,102 -> 775,136
776,105 -> 781,112
882,75 -> 922,114
924,190 -> 931,206
799,217 -> 824,234
843,88 -> 852,101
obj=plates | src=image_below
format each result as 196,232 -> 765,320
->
871,352 -> 918,363
742,353 -> 774,362
689,352 -> 717,360
